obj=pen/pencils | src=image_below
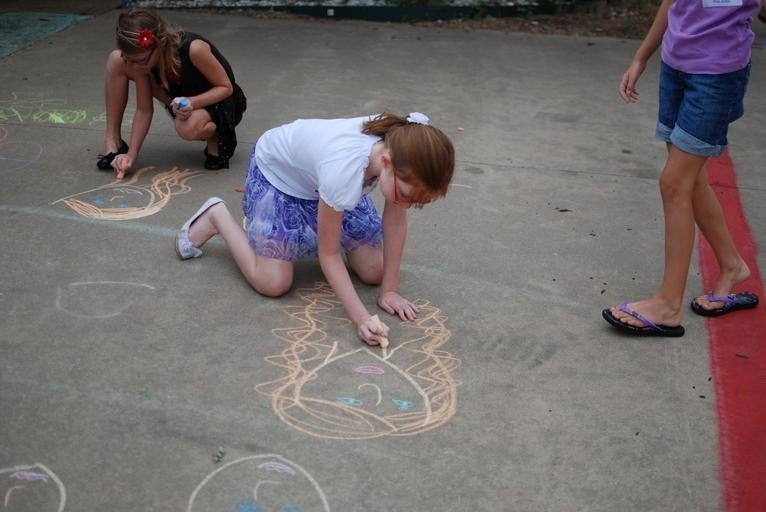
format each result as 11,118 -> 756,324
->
113,156 -> 130,180
370,314 -> 390,349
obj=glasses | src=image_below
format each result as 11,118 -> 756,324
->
120,46 -> 158,66
393,166 -> 424,209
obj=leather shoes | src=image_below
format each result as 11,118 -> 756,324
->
204,152 -> 222,169
174,196 -> 229,260
97,139 -> 128,170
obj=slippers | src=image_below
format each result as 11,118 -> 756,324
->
691,291 -> 760,318
602,301 -> 685,337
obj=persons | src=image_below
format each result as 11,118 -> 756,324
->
600,0 -> 761,337
174,108 -> 455,347
96,8 -> 247,174
